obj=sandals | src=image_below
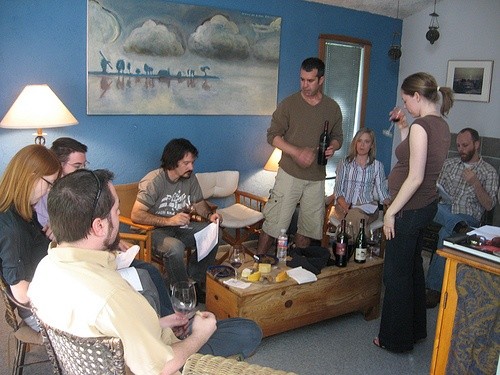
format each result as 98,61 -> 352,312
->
373,337 -> 410,355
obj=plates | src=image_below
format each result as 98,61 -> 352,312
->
208,265 -> 235,279
255,254 -> 279,265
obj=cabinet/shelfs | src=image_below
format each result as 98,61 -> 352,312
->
429,246 -> 500,375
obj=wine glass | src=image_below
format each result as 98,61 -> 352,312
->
381,106 -> 403,137
170,281 -> 197,340
229,245 -> 245,286
367,229 -> 380,260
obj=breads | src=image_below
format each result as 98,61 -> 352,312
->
275,270 -> 290,283
247,271 -> 261,282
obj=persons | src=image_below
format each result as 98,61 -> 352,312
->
257,57 -> 343,256
328,128 -> 391,246
33,136 -> 175,318
372,72 -> 455,354
130,138 -> 223,307
27,169 -> 264,375
425,128 -> 499,309
0,144 -> 161,333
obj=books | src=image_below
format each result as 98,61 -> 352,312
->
442,234 -> 500,264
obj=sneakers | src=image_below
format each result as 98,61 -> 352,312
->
425,289 -> 441,309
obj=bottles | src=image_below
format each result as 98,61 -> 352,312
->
335,219 -> 349,267
354,219 -> 367,263
317,120 -> 331,166
277,228 -> 288,263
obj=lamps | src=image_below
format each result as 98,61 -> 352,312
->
1,83 -> 79,146
263,147 -> 283,172
425,0 -> 440,44
388,0 -> 401,64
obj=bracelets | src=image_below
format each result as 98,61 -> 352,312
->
207,211 -> 213,220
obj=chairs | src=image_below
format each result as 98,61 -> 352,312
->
325,194 -> 389,257
0,275 -> 53,375
193,170 -> 268,265
110,183 -> 191,281
424,208 -> 494,264
32,312 -> 126,375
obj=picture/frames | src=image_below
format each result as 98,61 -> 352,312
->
445,58 -> 494,103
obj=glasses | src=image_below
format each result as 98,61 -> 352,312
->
76,167 -> 101,227
67,159 -> 91,171
43,179 -> 57,191
